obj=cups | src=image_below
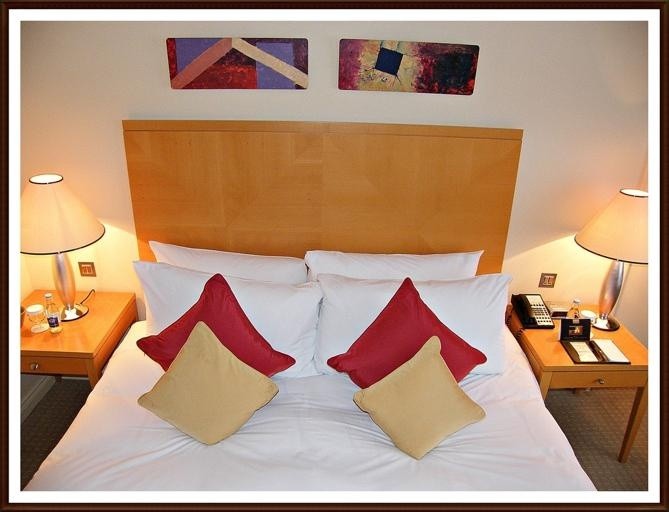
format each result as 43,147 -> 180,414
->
581,310 -> 597,326
20,304 -> 25,328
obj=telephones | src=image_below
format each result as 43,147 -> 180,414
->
511,294 -> 555,329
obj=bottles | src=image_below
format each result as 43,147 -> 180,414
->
44,293 -> 62,333
566,299 -> 580,319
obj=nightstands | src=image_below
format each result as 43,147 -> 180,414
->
508,305 -> 648,463
22,289 -> 139,390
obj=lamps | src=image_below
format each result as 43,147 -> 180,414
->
574,190 -> 647,332
22,173 -> 105,322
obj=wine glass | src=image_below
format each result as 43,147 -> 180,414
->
26,304 -> 48,334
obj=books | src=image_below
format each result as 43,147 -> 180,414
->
560,339 -> 631,365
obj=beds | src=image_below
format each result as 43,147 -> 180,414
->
22,119 -> 599,492
